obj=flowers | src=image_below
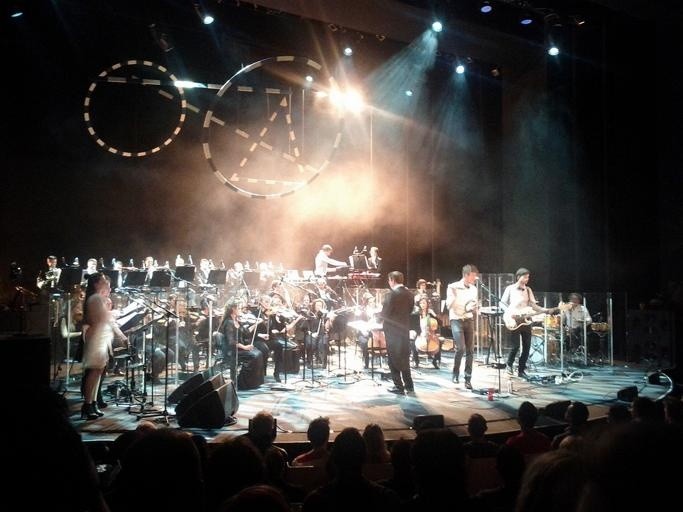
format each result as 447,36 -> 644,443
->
113,287 -> 128,296
474,276 -> 485,287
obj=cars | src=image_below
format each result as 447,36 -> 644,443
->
487,388 -> 494,401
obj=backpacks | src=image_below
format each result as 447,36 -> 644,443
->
591,322 -> 610,332
526,327 -> 559,365
542,315 -> 561,331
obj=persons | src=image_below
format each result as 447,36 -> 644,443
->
375,270 -> 416,394
249,245 -> 348,383
359,278 -> 442,369
293,392 -> 680,510
558,292 -> 592,354
220,302 -> 264,391
445,263 -> 480,390
2,385 -> 293,511
36,254 -> 246,289
80,272 -> 129,422
499,269 -> 555,381
132,294 -> 220,384
360,247 -> 383,287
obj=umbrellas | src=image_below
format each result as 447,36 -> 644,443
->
464,381 -> 472,389
505,364 -> 513,375
387,387 -> 405,394
452,375 -> 459,383
518,371 -> 527,378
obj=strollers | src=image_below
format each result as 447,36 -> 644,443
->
517,286 -> 524,291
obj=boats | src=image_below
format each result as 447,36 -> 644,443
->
167,365 -> 239,429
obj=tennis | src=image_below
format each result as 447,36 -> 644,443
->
367,329 -> 388,357
414,298 -> 440,355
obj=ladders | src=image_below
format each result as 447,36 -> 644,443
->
184,309 -> 200,319
213,308 -> 224,316
271,306 -> 306,319
237,313 -> 260,323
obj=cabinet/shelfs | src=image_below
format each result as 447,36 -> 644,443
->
507,378 -> 513,393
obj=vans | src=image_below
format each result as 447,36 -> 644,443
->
93,401 -> 103,417
80,403 -> 98,420
